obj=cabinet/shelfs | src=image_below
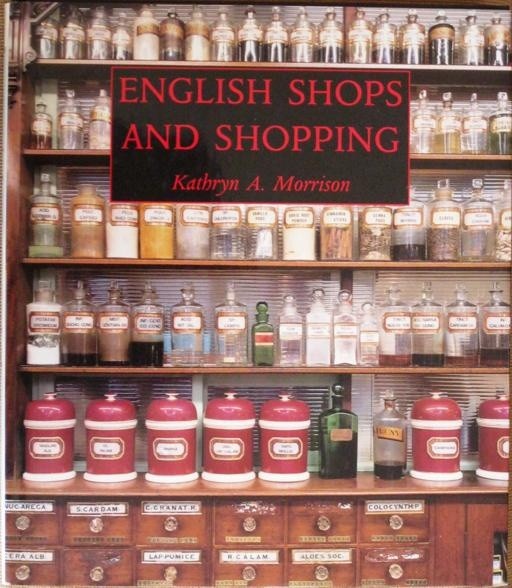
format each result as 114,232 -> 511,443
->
5,495 -> 511,588
6,1 -> 512,495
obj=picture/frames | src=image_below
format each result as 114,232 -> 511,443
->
109,67 -> 412,207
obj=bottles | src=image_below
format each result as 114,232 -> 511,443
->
25,3 -> 511,488
